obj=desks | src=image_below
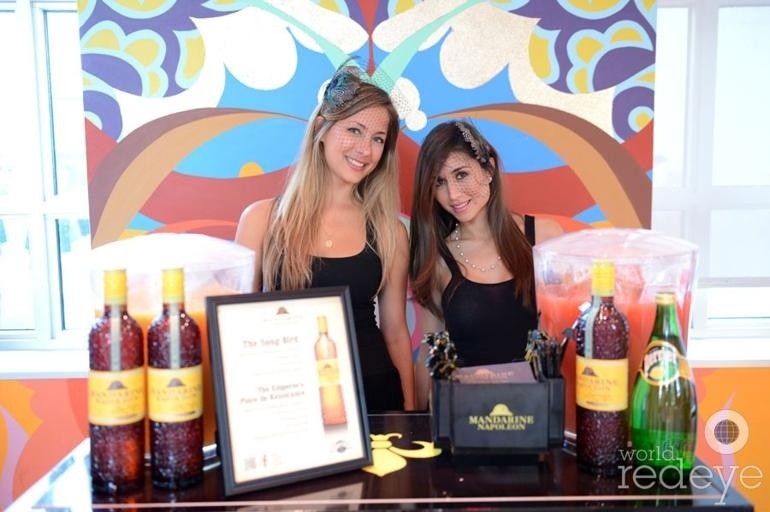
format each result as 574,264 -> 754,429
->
1,422 -> 758,510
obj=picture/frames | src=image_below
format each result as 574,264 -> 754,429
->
204,283 -> 376,497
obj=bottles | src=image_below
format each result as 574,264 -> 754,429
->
629,291 -> 699,481
574,258 -> 631,477
313,313 -> 347,428
144,266 -> 206,490
87,268 -> 146,496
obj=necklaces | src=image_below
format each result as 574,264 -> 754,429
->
454,221 -> 503,274
314,195 -> 359,251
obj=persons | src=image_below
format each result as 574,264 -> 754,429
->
407,120 -> 571,413
233,62 -> 417,413
